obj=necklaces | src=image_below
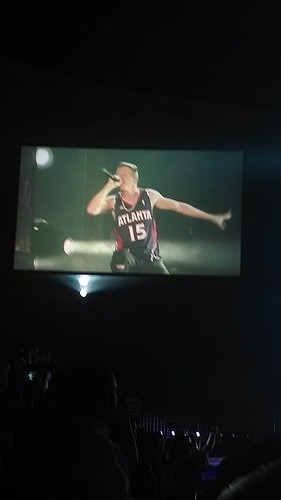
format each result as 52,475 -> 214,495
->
122,190 -> 139,212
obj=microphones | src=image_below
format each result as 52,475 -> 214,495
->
106,171 -> 119,182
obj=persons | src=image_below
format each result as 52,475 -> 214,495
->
216,432 -> 281,500
87,162 -> 232,275
0,333 -> 212,500
